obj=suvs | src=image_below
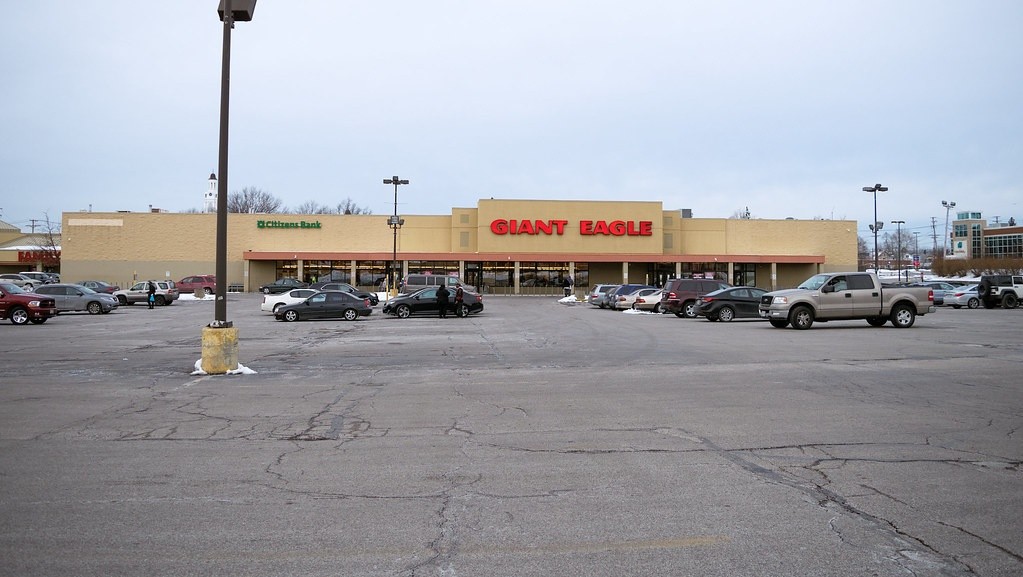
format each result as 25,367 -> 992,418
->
661,278 -> 740,318
175,275 -> 217,294
978,275 -> 1023,310
113,279 -> 180,306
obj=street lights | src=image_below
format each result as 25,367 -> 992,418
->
200,0 -> 258,374
891,221 -> 905,281
382,174 -> 409,296
942,201 -> 956,261
862,184 -> 888,275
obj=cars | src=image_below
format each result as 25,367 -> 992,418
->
261,281 -> 379,322
259,277 -> 306,294
383,286 -> 484,319
588,282 -> 666,313
692,286 -> 771,322
889,282 -> 980,308
0,271 -> 120,325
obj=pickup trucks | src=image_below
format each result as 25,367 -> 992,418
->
758,272 -> 936,330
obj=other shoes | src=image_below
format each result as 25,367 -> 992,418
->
147,306 -> 154,309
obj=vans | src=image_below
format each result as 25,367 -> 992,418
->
401,274 -> 476,295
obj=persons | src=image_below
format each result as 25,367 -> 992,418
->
451,283 -> 463,318
825,279 -> 840,286
146,280 -> 155,309
436,284 -> 450,319
535,277 -> 555,287
562,275 -> 574,297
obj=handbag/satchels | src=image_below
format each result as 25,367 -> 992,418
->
150,294 -> 155,301
